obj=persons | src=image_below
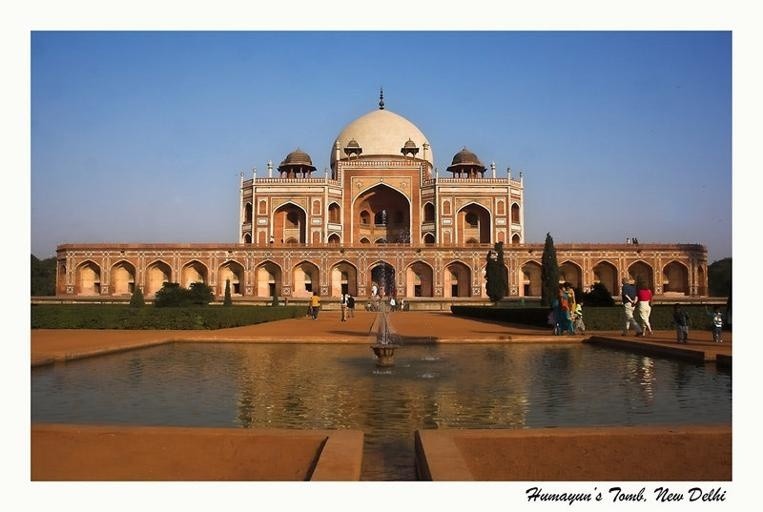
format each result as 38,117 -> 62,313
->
346,294 -> 355,318
624,237 -> 638,244
364,285 -> 405,312
621,277 -> 643,337
672,303 -> 691,345
308,291 -> 320,320
635,279 -> 654,336
710,313 -> 717,342
712,312 -> 722,342
340,293 -> 348,322
548,282 -> 586,336
